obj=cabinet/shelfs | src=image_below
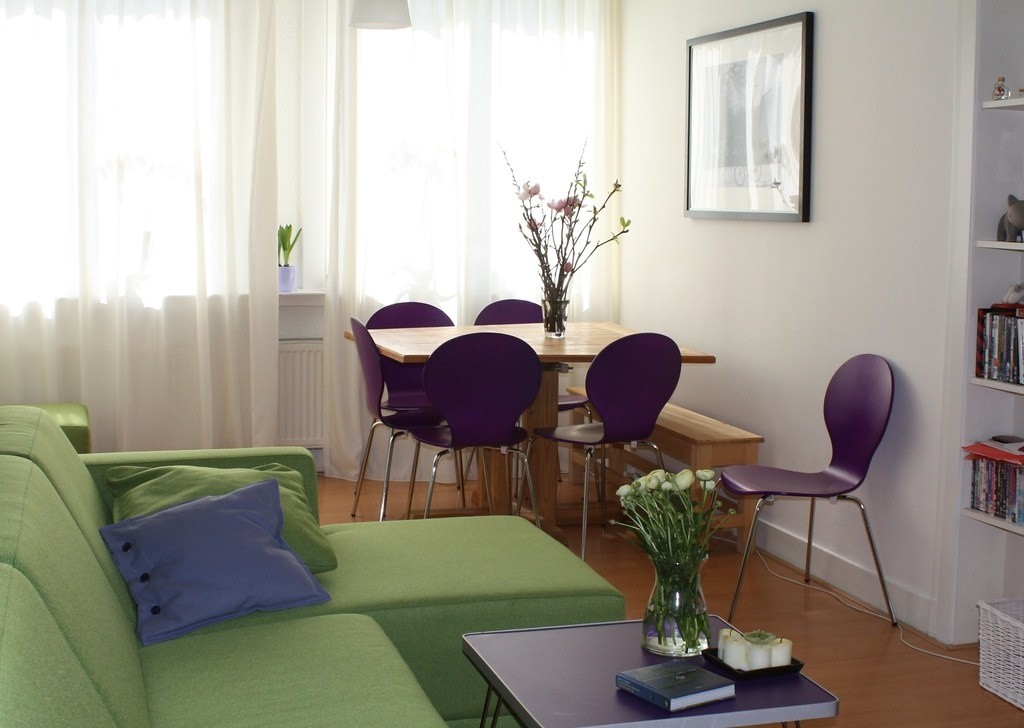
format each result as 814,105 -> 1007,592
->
962,95 -> 1024,537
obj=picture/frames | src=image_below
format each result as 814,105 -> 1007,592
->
683,10 -> 816,223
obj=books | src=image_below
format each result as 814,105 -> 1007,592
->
615,658 -> 736,712
975,307 -> 1024,385
961,441 -> 1024,527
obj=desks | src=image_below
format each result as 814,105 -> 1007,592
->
367,321 -> 717,528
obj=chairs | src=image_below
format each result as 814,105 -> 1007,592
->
720,354 -> 898,629
514,332 -> 682,564
409,333 -> 546,518
474,296 -> 604,506
350,316 -> 472,523
366,301 -> 468,510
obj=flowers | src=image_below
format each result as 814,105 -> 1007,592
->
498,135 -> 633,335
743,628 -> 776,646
609,465 -> 737,647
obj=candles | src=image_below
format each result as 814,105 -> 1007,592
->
746,641 -> 772,670
770,632 -> 793,667
718,629 -> 741,660
723,634 -> 747,668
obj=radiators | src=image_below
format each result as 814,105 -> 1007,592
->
275,339 -> 325,449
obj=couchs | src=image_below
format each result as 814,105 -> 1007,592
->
0,404 -> 626,728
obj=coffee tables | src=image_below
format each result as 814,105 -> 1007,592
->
460,614 -> 840,728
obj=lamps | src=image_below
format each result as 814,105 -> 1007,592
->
348,0 -> 413,31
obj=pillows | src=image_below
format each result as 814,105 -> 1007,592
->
98,479 -> 332,648
103,462 -> 338,575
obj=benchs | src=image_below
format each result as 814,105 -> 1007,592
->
566,384 -> 765,538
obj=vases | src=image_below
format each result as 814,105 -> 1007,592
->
540,289 -> 570,338
640,550 -> 710,654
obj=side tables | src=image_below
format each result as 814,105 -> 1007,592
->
25,404 -> 91,454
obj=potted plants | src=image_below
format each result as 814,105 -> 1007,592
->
275,224 -> 304,294
